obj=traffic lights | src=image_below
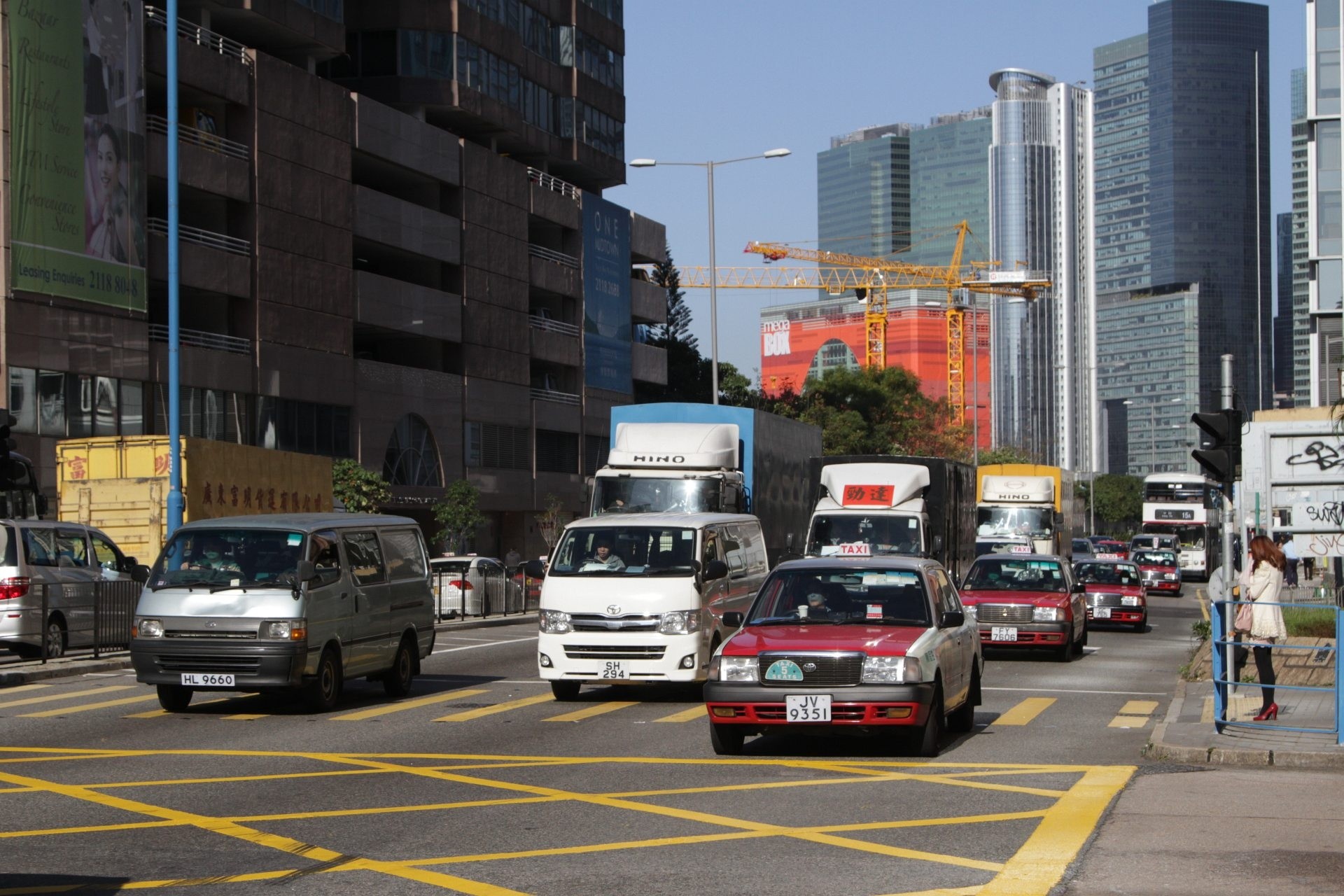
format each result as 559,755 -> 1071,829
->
1185,409 -> 1242,482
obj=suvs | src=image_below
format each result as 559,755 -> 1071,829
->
1072,536 -> 1099,575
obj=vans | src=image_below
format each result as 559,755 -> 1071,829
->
130,511 -> 436,711
1,518 -> 150,662
538,510 -> 771,700
1130,534 -> 1183,586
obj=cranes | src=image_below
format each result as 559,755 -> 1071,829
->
649,217 -> 1052,459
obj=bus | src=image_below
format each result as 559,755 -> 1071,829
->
1141,472 -> 1225,579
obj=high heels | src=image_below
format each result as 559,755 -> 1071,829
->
1253,702 -> 1279,720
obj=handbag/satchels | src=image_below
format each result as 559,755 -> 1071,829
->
1233,588 -> 1253,634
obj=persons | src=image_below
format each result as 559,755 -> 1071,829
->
791,578 -> 832,618
1282,534 -> 1302,587
605,486 -> 635,513
85,122 -> 140,264
1232,527 -> 1288,581
1304,557 -> 1316,580
62,541 -> 82,565
1078,566 -> 1097,582
581,537 -> 624,566
875,528 -> 896,545
180,539 -> 240,572
506,546 -> 520,567
825,522 -> 845,544
980,565 -> 1005,586
277,539 -> 324,585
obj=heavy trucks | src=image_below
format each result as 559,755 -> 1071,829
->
590,402 -> 823,583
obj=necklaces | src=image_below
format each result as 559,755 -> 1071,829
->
1236,536 -> 1288,720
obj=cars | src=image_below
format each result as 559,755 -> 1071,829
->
955,546 -> 1090,661
1093,544 -> 1111,554
1099,540 -> 1129,561
1130,548 -> 1188,597
703,541 -> 985,754
1070,553 -> 1154,632
511,555 -> 548,604
1087,536 -> 1116,544
429,555 -> 523,619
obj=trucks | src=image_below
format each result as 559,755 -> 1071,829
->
803,456 -> 978,620
56,434 -> 335,575
974,464 -> 1074,571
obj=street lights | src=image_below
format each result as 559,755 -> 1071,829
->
1122,397 -> 1182,474
629,147 -> 793,406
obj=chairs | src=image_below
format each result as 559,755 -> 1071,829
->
57,543 -> 83,567
224,543 -> 254,579
822,581 -> 853,620
611,538 -> 641,566
